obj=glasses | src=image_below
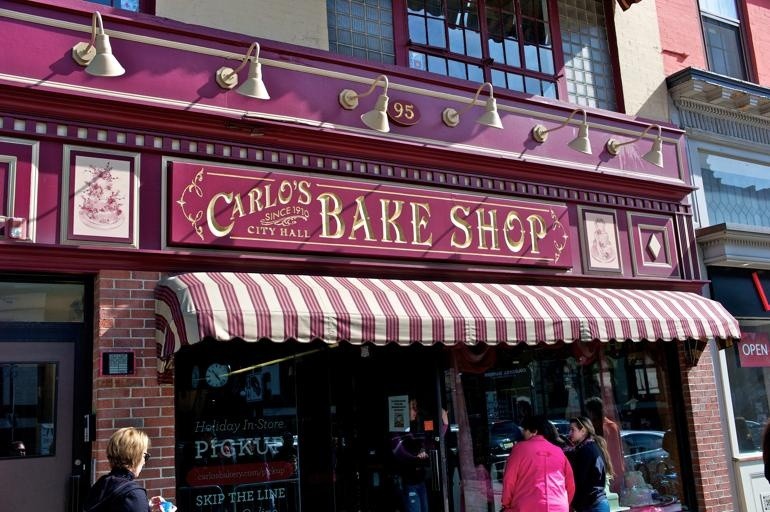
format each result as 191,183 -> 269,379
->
143,453 -> 152,461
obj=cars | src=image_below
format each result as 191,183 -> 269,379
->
744,418 -> 762,450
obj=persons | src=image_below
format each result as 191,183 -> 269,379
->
8,441 -> 27,457
80,426 -> 169,512
363,394 -> 429,512
503,396 -> 627,512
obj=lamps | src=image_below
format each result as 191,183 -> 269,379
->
215,41 -> 272,100
338,74 -> 392,135
531,109 -> 592,156
72,10 -> 127,77
442,82 -> 504,129
607,123 -> 665,169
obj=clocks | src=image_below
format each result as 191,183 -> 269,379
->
191,360 -> 230,389
